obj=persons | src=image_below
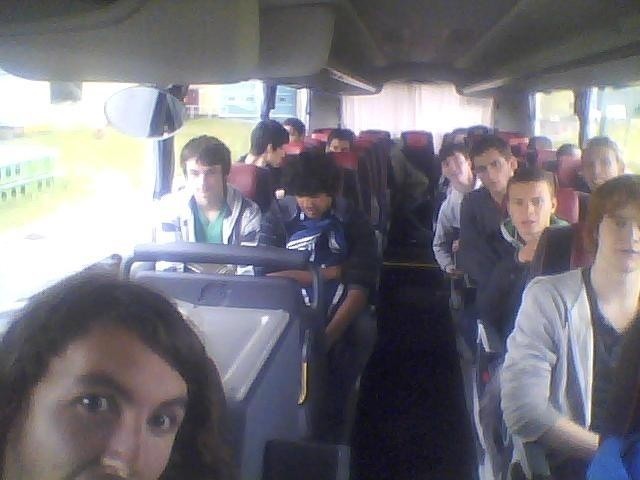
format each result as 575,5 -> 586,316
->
432,109 -> 625,412
237,119 -> 290,198
250,151 -> 375,356
586,296 -> 640,480
0,260 -> 231,479
281,117 -> 305,145
499,174 -> 640,480
325,129 -> 352,153
153,134 -> 262,276
380,133 -> 432,248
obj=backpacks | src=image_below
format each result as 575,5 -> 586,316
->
284,209 -> 350,320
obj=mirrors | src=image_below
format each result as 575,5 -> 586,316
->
103,86 -> 188,139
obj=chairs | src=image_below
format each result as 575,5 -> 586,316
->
398,129 -> 437,192
440,129 -> 624,480
120,129 -> 392,476
